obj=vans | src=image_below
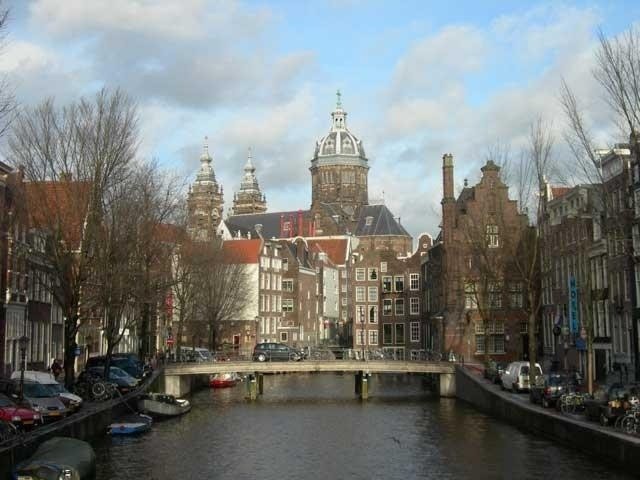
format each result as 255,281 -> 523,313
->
166,346 -> 218,363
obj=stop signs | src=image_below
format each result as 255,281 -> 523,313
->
167,339 -> 174,345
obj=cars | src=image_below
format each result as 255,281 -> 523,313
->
484,359 -> 639,437
0,352 -> 151,444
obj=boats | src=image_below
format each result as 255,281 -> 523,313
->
14,437 -> 97,480
209,373 -> 238,387
139,393 -> 191,417
107,411 -> 153,436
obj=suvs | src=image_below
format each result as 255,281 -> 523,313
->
252,342 -> 303,361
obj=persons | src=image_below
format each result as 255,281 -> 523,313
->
50,359 -> 63,380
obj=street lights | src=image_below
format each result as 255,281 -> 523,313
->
553,325 -> 590,411
254,316 -> 259,345
360,313 -> 367,361
